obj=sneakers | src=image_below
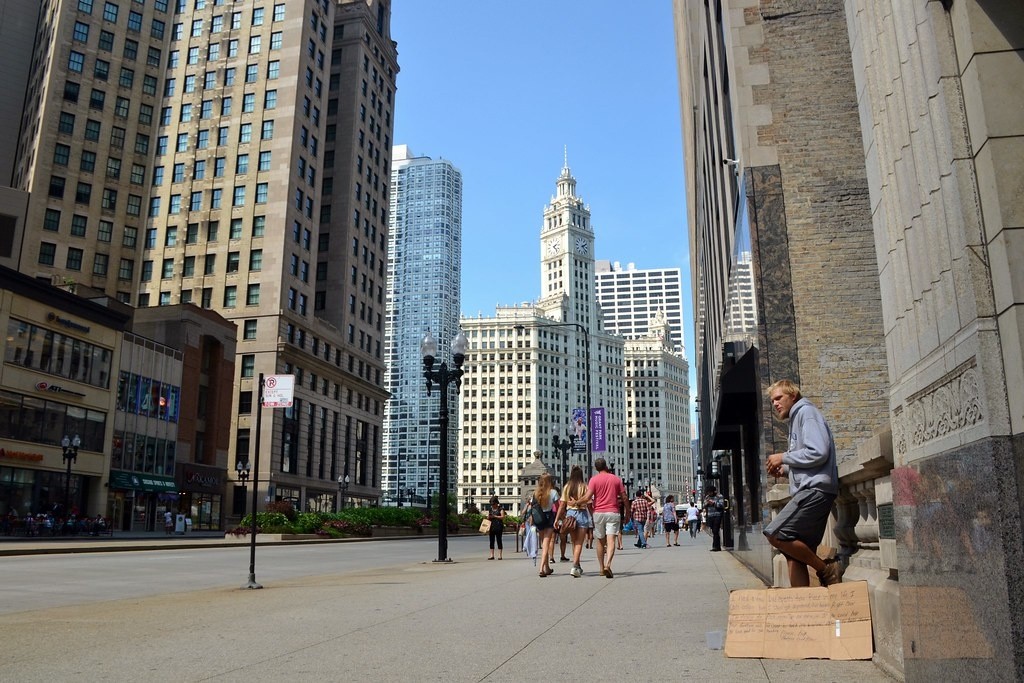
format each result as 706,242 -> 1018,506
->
816,556 -> 844,587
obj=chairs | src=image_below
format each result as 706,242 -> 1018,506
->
0,516 -> 114,537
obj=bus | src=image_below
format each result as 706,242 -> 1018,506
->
675,504 -> 692,529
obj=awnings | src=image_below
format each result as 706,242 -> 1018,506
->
109,470 -> 186,494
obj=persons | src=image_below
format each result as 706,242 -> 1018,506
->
703,485 -> 724,552
486,496 -> 504,561
571,415 -> 587,441
762,379 -> 839,588
9,506 -> 106,536
522,458 -> 706,578
163,508 -> 173,535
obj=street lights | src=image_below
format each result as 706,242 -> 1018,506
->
487,465 -> 494,496
551,419 -> 576,488
513,324 -> 592,480
337,475 -> 349,510
621,469 -> 634,500
62,434 -> 80,535
666,447 -> 695,503
427,429 -> 463,510
421,325 -> 469,562
408,484 -> 416,507
612,424 -> 652,497
237,461 -> 250,521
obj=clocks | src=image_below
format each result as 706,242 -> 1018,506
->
575,237 -> 588,255
547,237 -> 560,255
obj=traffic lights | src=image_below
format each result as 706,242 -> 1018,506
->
692,490 -> 695,496
647,492 -> 652,498
465,496 -> 468,505
471,497 -> 474,505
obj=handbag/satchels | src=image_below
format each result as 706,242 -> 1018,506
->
560,515 -> 577,534
518,523 -> 526,536
531,492 -> 552,531
479,519 -> 492,534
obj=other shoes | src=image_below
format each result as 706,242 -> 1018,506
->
545,568 -> 553,575
570,567 -> 580,577
539,571 -> 547,577
498,557 -> 502,560
710,547 -> 721,551
560,556 -> 569,561
585,543 -> 589,549
549,559 -> 555,563
667,544 -> 671,547
590,546 -> 593,549
674,542 -> 679,546
488,556 -> 494,560
634,543 -> 641,548
617,547 -> 623,550
575,567 -> 583,574
604,566 -> 613,578
600,569 -> 605,576
642,544 -> 646,548
